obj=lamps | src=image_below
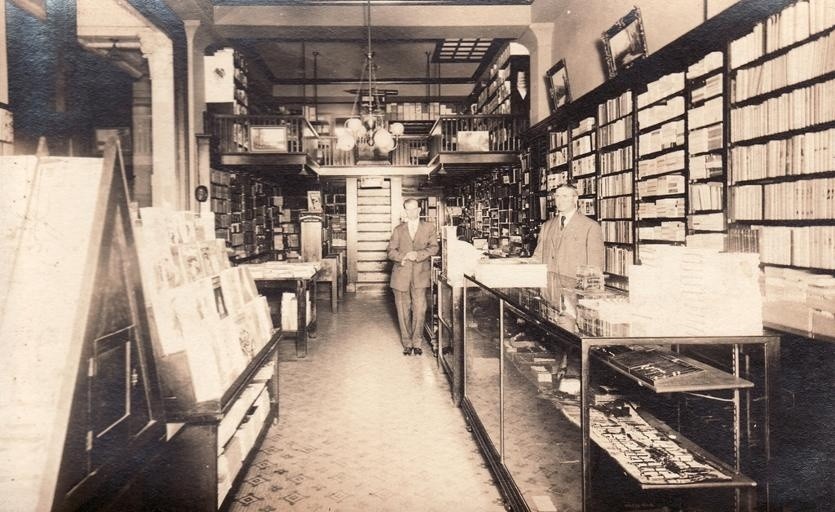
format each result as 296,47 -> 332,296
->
336,1 -> 405,153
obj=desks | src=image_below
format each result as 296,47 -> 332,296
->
251,246 -> 347,357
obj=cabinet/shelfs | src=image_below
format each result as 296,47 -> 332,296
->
206,41 -> 301,266
165,327 -> 283,511
420,1 -> 835,512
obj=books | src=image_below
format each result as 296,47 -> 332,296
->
590,346 -> 704,386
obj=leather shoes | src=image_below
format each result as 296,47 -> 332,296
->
403,346 -> 413,356
414,346 -> 422,354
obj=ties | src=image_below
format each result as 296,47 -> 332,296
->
560,216 -> 565,232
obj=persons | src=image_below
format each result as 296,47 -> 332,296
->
386,198 -> 439,355
530,185 -> 604,280
